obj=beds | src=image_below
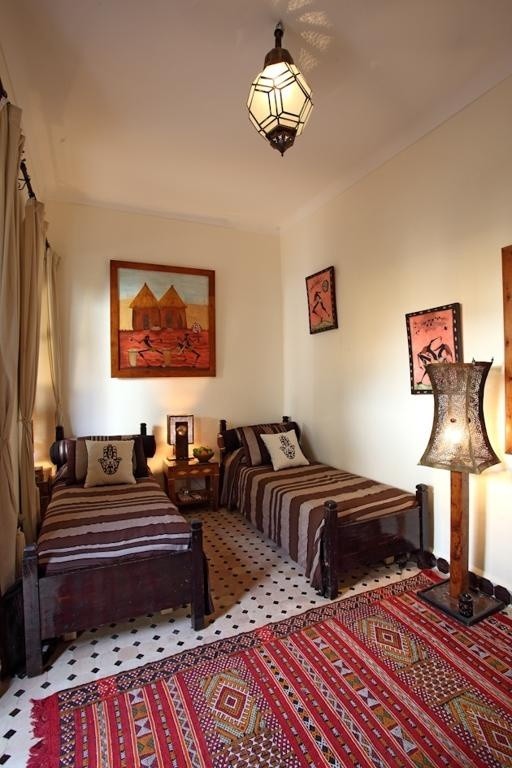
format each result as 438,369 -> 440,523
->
21,423 -> 215,679
220,416 -> 434,601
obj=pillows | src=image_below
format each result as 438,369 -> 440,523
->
259,429 -> 311,472
75,437 -> 135,487
85,439 -> 138,488
237,423 -> 287,467
65,436 -> 147,486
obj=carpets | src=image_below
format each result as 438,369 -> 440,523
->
26,572 -> 511,767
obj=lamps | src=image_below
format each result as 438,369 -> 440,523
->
245,21 -> 313,158
416,359 -> 508,625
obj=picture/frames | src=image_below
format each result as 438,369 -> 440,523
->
168,414 -> 194,444
305,265 -> 337,335
501,245 -> 511,457
110,260 -> 216,378
406,302 -> 463,394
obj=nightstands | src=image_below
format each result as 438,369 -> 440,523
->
34,467 -> 51,511
162,460 -> 219,512
